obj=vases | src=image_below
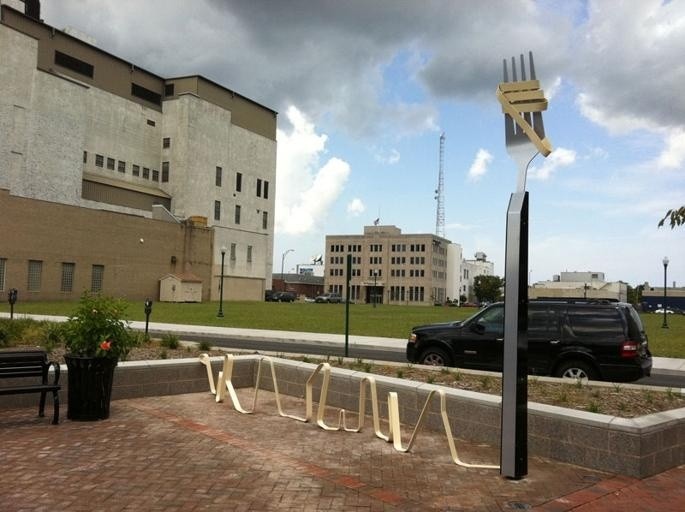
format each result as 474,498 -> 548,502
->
63,355 -> 118,422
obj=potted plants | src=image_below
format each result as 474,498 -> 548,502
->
61,288 -> 145,362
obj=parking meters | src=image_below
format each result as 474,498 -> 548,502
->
144,297 -> 153,337
8,287 -> 18,318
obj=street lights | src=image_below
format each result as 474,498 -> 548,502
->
281,249 -> 296,290
373,269 -> 378,309
216,246 -> 227,318
661,255 -> 670,329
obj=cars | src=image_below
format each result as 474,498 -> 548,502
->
314,292 -> 342,304
405,295 -> 652,385
656,308 -> 675,315
267,291 -> 296,303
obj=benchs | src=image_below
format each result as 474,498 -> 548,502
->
0,350 -> 62,425
